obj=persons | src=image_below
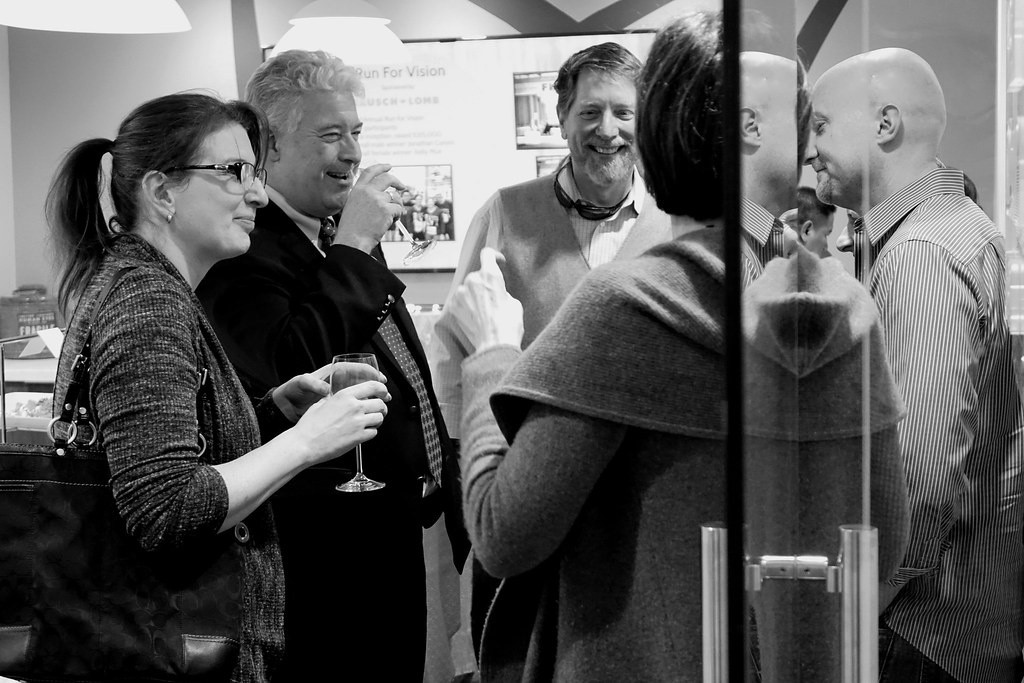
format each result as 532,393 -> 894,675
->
30,93 -> 392,683
196,48 -> 453,683
736,47 -> 802,298
797,186 -> 837,259
430,42 -> 671,682
802,45 -> 1022,683
385,185 -> 457,241
443,8 -> 914,683
837,157 -> 948,264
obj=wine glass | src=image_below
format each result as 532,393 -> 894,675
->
330,354 -> 386,493
346,160 -> 437,266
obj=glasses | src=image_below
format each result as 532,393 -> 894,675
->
554,162 -> 631,221
164,162 -> 268,189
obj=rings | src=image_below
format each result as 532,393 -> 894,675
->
385,188 -> 396,203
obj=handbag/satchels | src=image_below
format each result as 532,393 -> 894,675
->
0,266 -> 240,683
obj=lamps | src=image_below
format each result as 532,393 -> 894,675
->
265,0 -> 415,66
0,0 -> 193,35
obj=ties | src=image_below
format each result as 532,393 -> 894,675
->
318,219 -> 442,487
